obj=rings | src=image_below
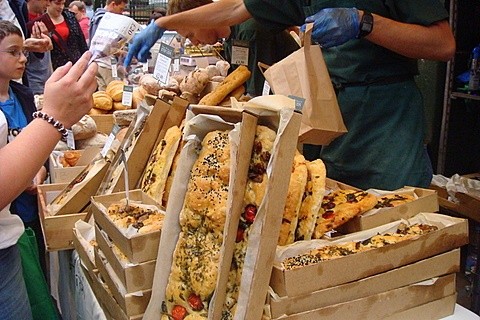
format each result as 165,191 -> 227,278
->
46,46 -> 49,51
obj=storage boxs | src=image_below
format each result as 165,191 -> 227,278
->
28,64 -> 468,320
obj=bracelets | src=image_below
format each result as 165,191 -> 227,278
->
32,112 -> 69,137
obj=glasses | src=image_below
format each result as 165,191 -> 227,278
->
0,49 -> 29,58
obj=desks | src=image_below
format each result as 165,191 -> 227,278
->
57,247 -> 480,320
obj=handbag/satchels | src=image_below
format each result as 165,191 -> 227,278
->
258,22 -> 348,145
17,227 -> 64,320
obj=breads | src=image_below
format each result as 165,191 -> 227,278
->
48,59 -> 438,320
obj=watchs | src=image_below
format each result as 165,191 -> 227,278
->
359,10 -> 374,42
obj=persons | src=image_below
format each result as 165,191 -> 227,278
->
124,0 -> 455,191
0,0 -> 127,320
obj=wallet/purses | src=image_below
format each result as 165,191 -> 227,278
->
26,13 -> 56,35
